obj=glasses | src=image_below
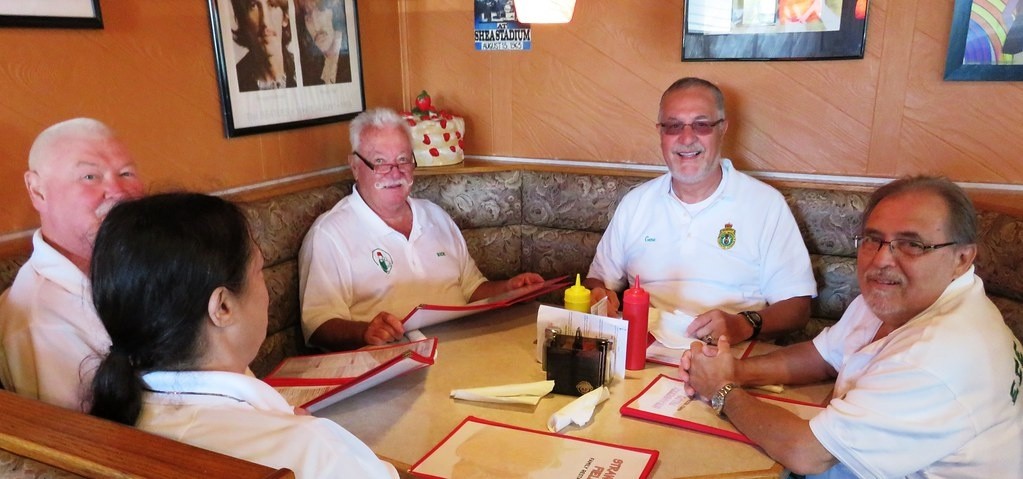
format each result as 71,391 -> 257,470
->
660,118 -> 724,135
352,151 -> 418,175
853,235 -> 954,258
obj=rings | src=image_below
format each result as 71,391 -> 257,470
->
705,335 -> 712,344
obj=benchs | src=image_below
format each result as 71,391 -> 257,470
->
307,302 -> 834,478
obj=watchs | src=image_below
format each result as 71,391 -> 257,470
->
736,310 -> 763,341
712,382 -> 741,419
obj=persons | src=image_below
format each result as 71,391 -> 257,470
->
299,105 -> 544,352
671,172 -> 1023,479
61,189 -> 400,479
227,0 -> 351,92
576,76 -> 818,346
0,116 -> 142,417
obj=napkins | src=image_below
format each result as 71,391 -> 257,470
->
548,385 -> 610,433
449,380 -> 555,406
645,307 -> 707,349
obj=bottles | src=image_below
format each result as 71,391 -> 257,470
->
622,274 -> 650,371
541,327 -> 562,371
563,273 -> 591,314
604,340 -> 614,385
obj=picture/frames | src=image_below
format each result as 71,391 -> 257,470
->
681,0 -> 870,62
0,0 -> 105,30
206,0 -> 365,139
944,0 -> 1023,82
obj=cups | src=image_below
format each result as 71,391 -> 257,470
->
731,0 -> 744,26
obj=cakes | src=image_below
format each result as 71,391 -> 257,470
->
396,89 -> 465,167
